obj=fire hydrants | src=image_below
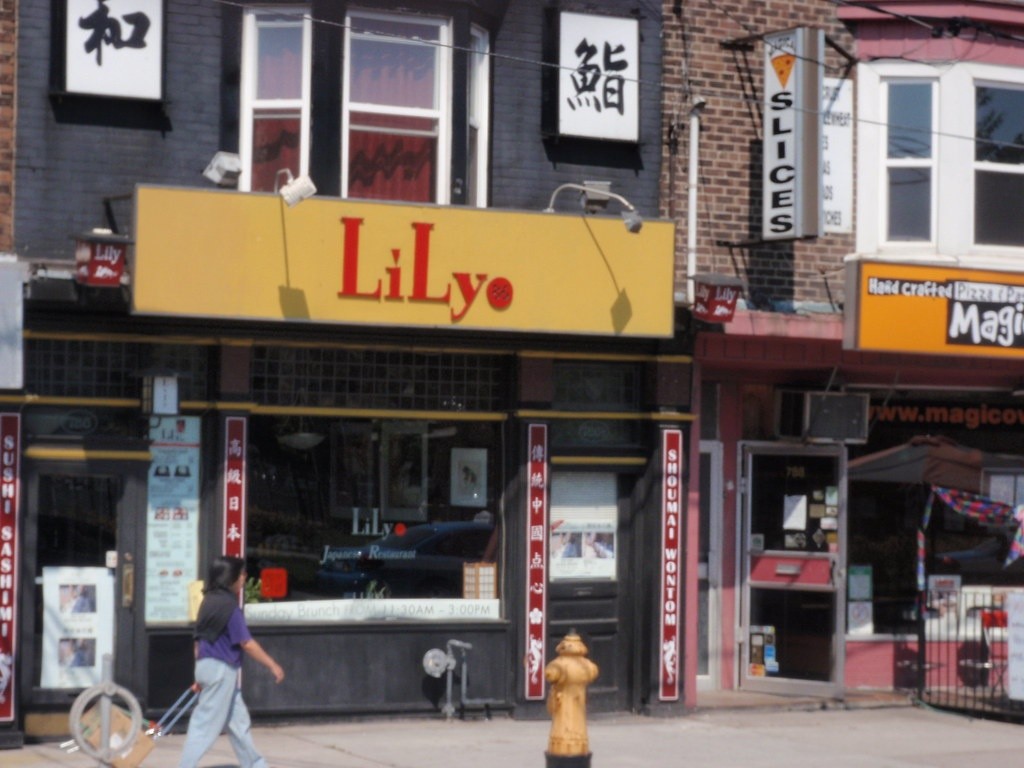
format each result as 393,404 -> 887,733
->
544,627 -> 600,768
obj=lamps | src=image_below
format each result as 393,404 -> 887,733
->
276,377 -> 323,449
202,151 -> 241,188
582,180 -> 612,214
275,166 -> 317,204
546,183 -> 642,233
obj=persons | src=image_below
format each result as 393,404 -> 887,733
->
179,556 -> 284,768
61,585 -> 95,613
60,638 -> 87,667
555,532 -> 581,557
582,532 -> 613,558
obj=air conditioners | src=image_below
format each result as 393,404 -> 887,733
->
774,390 -> 869,444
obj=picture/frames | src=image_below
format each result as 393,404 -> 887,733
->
327,421 -> 430,519
451,447 -> 487,506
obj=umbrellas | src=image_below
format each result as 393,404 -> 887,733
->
843,433 -> 995,491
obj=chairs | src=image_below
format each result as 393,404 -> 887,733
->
982,606 -> 1009,696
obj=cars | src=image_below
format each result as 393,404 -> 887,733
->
317,517 -> 496,600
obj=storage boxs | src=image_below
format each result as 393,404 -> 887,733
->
82,698 -> 155,768
